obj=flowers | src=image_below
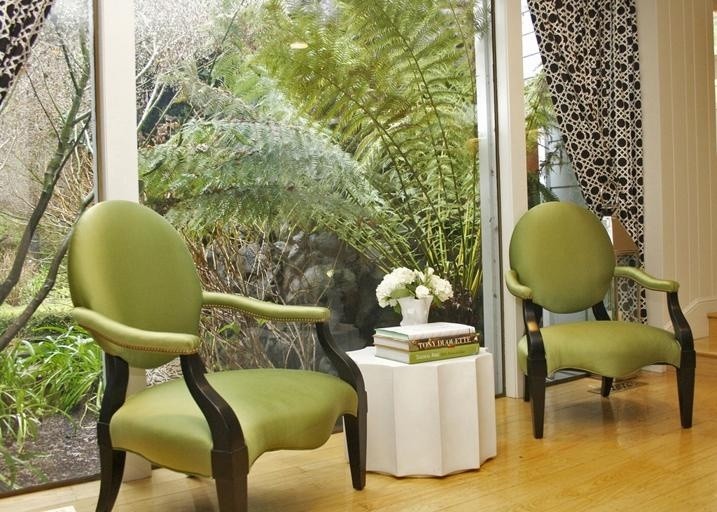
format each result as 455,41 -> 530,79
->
375,261 -> 454,313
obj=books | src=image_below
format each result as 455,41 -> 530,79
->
371,321 -> 480,364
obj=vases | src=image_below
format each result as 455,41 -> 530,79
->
399,297 -> 433,326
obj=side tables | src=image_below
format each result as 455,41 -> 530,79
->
339,345 -> 501,478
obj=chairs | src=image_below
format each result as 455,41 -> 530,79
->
67,200 -> 368,511
503,198 -> 697,441
